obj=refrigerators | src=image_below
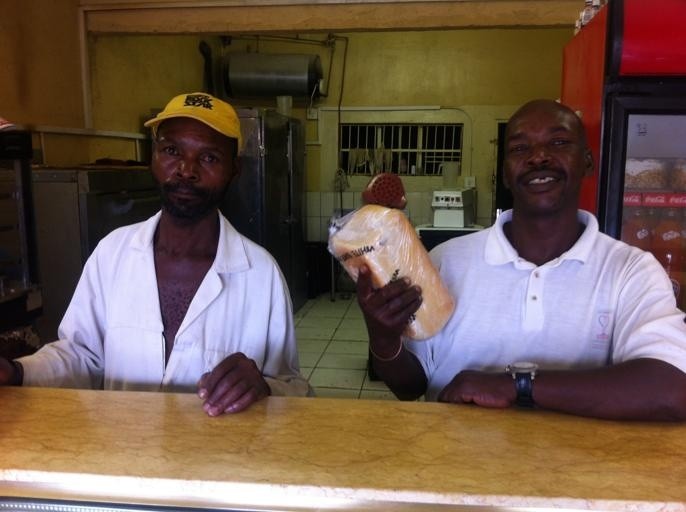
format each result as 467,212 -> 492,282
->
561,1 -> 685,318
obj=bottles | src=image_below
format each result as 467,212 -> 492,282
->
622,208 -> 686,309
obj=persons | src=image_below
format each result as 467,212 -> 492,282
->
0,91 -> 319,417
355,96 -> 686,422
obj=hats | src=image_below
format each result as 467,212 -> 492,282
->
144,91 -> 243,159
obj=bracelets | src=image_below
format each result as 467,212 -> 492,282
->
368,335 -> 403,363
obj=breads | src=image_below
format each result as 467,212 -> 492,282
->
326,203 -> 456,340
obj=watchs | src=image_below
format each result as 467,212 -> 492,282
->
506,360 -> 540,408
